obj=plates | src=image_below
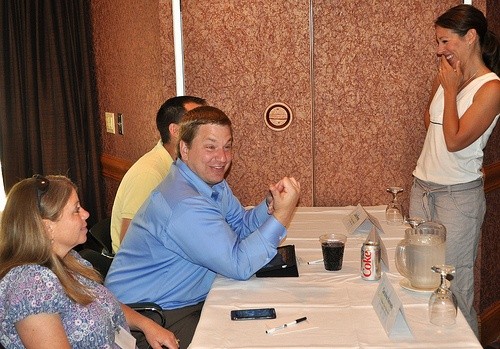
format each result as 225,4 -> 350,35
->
399,278 -> 451,293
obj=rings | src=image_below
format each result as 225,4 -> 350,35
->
438,69 -> 442,71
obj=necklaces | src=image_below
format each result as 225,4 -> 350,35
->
457,72 -> 476,91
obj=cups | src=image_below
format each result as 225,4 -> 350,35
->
319,233 -> 347,271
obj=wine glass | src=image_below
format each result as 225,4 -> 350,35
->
428,263 -> 458,326
384,187 -> 404,224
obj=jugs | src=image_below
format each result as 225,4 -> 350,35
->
394,222 -> 447,289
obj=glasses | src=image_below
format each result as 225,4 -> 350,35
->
33,173 -> 50,212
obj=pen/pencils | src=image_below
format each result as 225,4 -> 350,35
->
266,317 -> 307,334
307,259 -> 324,264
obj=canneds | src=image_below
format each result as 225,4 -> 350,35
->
360,242 -> 382,281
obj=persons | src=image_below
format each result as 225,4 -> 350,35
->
0,173 -> 182,349
110,96 -> 207,253
408,4 -> 500,337
104,106 -> 300,349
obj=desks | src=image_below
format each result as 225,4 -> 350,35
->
187,205 -> 484,349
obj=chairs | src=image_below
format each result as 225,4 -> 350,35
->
78,217 -> 165,349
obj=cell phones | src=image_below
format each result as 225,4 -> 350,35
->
231,308 -> 276,320
